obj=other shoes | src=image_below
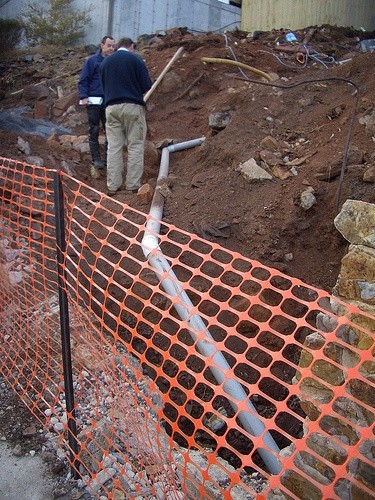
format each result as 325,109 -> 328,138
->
92,159 -> 105,169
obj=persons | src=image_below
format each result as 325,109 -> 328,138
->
99,37 -> 153,195
77,35 -> 115,169
